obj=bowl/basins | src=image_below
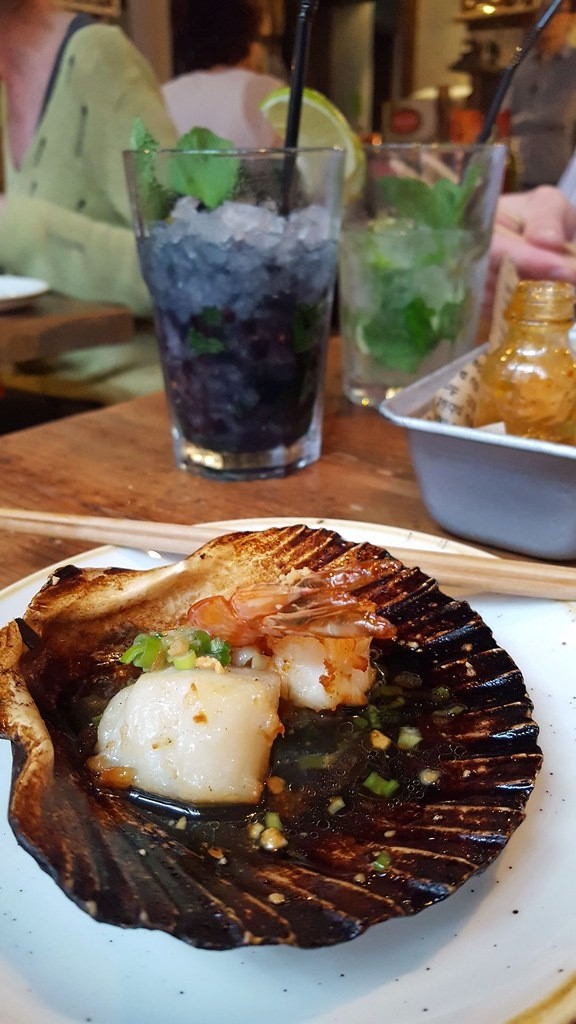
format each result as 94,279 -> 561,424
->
379,343 -> 576,560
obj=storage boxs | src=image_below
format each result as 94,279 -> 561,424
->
379,98 -> 438,142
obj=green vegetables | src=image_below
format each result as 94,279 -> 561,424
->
347,160 -> 482,375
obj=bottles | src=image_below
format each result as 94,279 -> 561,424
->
472,280 -> 576,446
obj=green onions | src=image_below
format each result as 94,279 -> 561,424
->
120,629 -> 232,672
249,674 -> 463,874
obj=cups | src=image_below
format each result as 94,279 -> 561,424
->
339,146 -> 506,408
124,148 -> 345,482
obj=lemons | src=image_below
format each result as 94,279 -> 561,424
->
259,85 -> 366,210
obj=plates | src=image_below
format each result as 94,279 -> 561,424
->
0,519 -> 575,1023
0,275 -> 50,312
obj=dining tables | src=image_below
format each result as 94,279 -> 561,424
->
0,290 -> 576,1024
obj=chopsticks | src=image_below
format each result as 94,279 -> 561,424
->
0,508 -> 576,603
